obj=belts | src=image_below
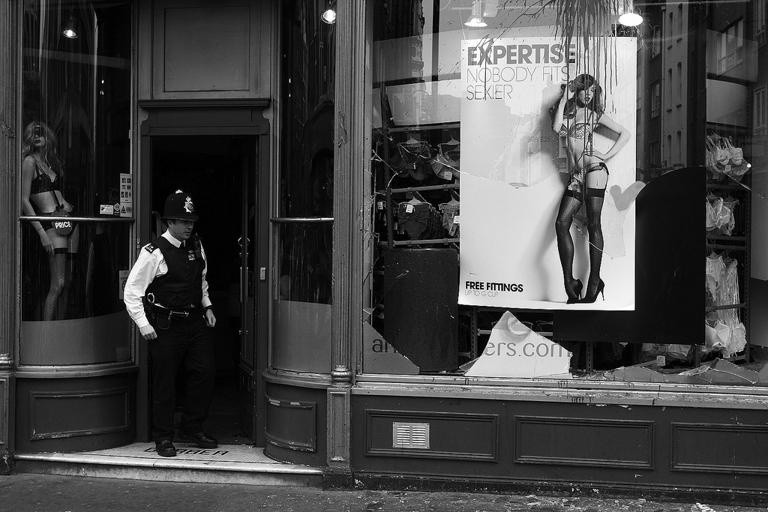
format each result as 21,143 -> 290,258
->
147,301 -> 198,319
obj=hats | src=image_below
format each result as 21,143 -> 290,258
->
159,189 -> 200,221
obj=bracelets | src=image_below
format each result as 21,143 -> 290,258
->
204,306 -> 212,316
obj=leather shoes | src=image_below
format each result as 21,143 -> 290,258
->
179,426 -> 218,449
154,436 -> 177,457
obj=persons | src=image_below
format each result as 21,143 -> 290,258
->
20,121 -> 80,321
122,190 -> 218,456
548,73 -> 630,304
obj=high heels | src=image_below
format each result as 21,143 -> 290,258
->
578,278 -> 606,303
566,278 -> 583,304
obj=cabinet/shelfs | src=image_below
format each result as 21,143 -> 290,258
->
685,73 -> 759,365
374,75 -> 593,371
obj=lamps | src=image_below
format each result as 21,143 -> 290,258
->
320,1 -> 336,24
61,17 -> 80,39
464,0 -> 488,28
617,3 -> 646,33
